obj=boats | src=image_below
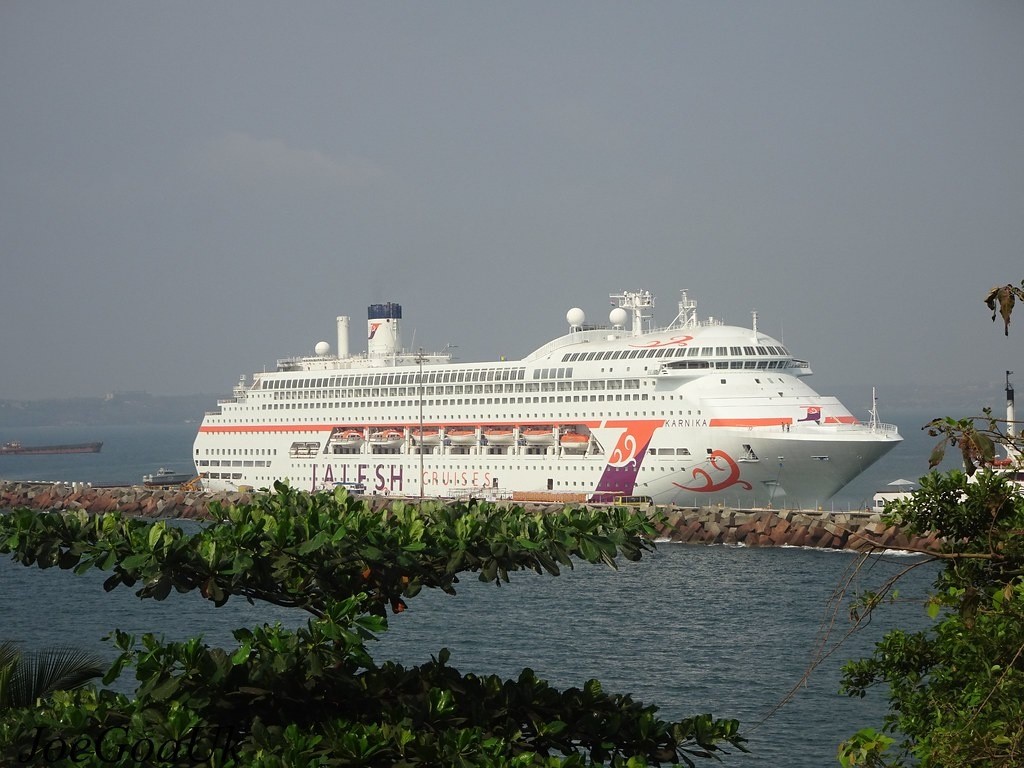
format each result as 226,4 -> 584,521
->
522,428 -> 554,447
411,431 -> 440,445
330,429 -> 363,448
447,430 -> 476,447
956,370 -> 1024,502
369,429 -> 404,449
483,429 -> 514,447
559,433 -> 589,451
872,478 -> 940,514
142,468 -> 194,486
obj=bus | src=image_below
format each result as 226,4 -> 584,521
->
331,482 -> 367,495
612,495 -> 653,507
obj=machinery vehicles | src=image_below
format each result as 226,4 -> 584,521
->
179,473 -> 206,491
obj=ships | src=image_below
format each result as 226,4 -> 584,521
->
191,287 -> 905,512
